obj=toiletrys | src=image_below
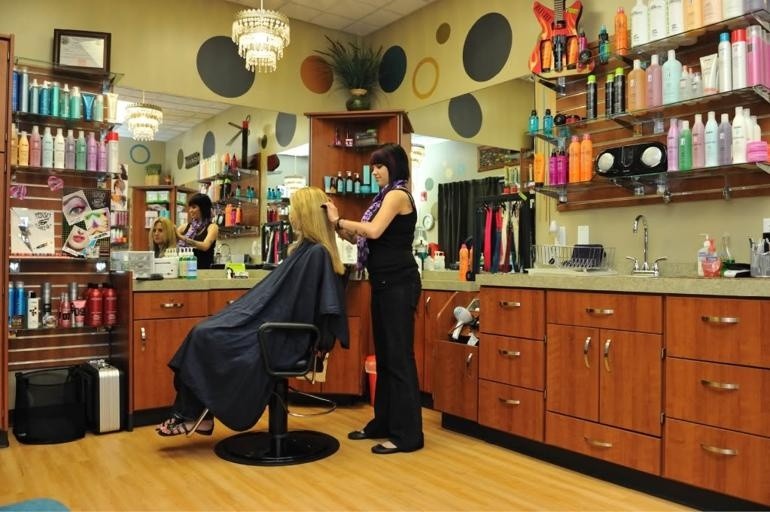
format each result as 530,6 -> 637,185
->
532,103 -> 770,190
527,26 -> 770,132
539,0 -> 768,70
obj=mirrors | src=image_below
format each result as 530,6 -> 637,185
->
415,76 -> 534,258
116,83 -> 309,270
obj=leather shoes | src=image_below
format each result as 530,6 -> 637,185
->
372,443 -> 402,453
348,430 -> 369,440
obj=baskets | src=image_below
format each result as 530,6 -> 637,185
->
529,243 -> 617,271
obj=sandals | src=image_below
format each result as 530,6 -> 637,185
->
155,412 -> 215,435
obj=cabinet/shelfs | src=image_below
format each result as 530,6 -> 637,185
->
544,288 -> 660,485
476,282 -> 545,447
3,33 -> 114,446
132,184 -> 199,253
135,286 -> 206,411
303,110 -> 414,216
527,9 -> 769,212
196,152 -> 262,238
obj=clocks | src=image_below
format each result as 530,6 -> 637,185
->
422,208 -> 435,234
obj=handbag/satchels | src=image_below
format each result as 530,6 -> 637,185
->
748,235 -> 769,278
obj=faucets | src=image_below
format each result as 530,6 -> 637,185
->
217,242 -> 233,263
632,214 -> 650,271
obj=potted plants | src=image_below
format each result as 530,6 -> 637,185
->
312,34 -> 385,110
143,162 -> 162,186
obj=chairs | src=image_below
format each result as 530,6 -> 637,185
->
215,260 -> 365,450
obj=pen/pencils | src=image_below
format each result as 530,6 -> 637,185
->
749,237 -> 770,276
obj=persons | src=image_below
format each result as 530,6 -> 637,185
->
153,186 -> 351,437
146,216 -> 177,258
67,228 -> 91,251
177,194 -> 220,269
111,181 -> 127,208
320,142 -> 425,456
63,196 -> 89,221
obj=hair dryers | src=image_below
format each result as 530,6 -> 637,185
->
452,305 -> 473,340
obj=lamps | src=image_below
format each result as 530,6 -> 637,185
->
124,91 -> 168,143
229,1 -> 294,74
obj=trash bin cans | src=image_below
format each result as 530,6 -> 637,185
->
365,355 -> 377,408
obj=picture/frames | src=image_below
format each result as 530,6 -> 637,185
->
49,26 -> 114,73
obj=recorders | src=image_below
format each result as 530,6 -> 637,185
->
595,141 -> 667,178
